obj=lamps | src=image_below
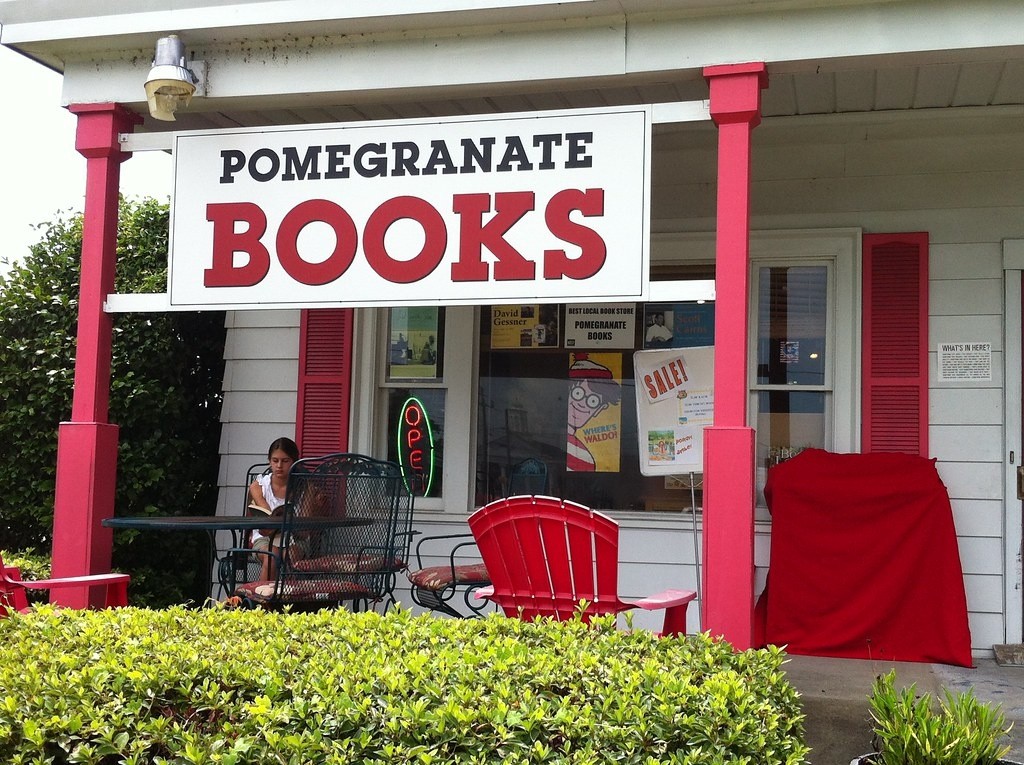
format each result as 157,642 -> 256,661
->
143,34 -> 205,122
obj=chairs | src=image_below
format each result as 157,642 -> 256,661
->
236,453 -> 402,614
0,553 -> 130,617
409,534 -> 498,620
467,494 -> 698,639
292,460 -> 422,617
217,463 -> 341,602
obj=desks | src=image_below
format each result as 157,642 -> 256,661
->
102,517 -> 379,611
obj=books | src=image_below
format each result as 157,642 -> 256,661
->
247,503 -> 295,517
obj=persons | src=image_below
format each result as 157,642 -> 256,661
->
647,313 -> 672,343
249,436 -> 318,583
